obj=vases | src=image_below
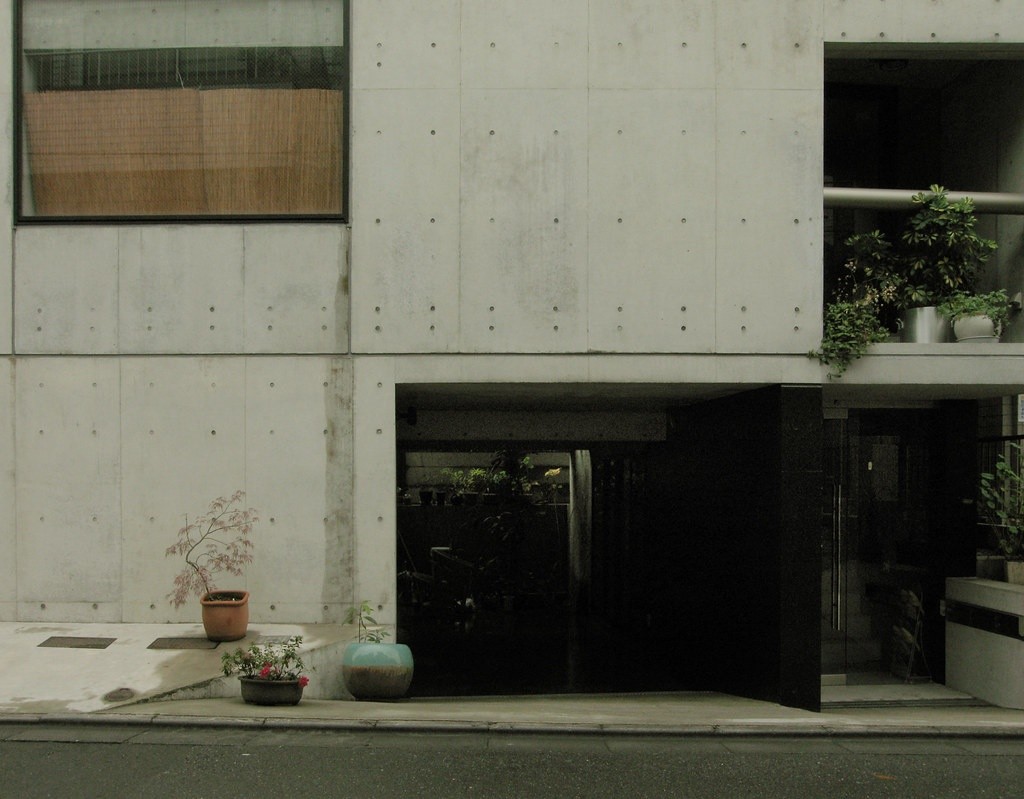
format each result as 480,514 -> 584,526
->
237,674 -> 304,707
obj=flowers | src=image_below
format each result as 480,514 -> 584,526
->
220,633 -> 311,689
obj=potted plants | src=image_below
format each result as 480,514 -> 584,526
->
163,489 -> 259,641
417,457 -> 561,507
961,441 -> 1024,586
340,598 -> 414,703
843,183 -> 999,344
935,286 -> 1012,343
